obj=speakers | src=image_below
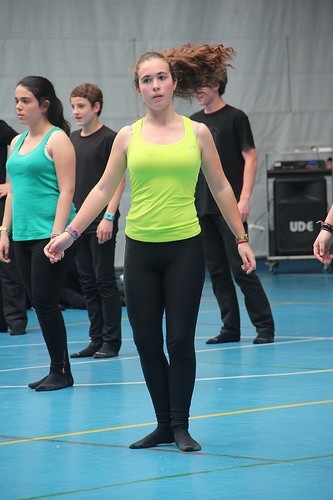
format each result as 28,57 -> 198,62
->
266,175 -> 333,274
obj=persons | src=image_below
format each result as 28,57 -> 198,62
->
312,203 -> 333,265
68,83 -> 125,358
0,76 -> 77,391
45,50 -> 256,451
0,119 -> 28,336
188,67 -> 275,344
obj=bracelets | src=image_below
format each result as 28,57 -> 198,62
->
64,225 -> 81,240
320,223 -> 332,233
0,225 -> 11,235
235,234 -> 248,245
50,233 -> 62,238
103,212 -> 116,221
